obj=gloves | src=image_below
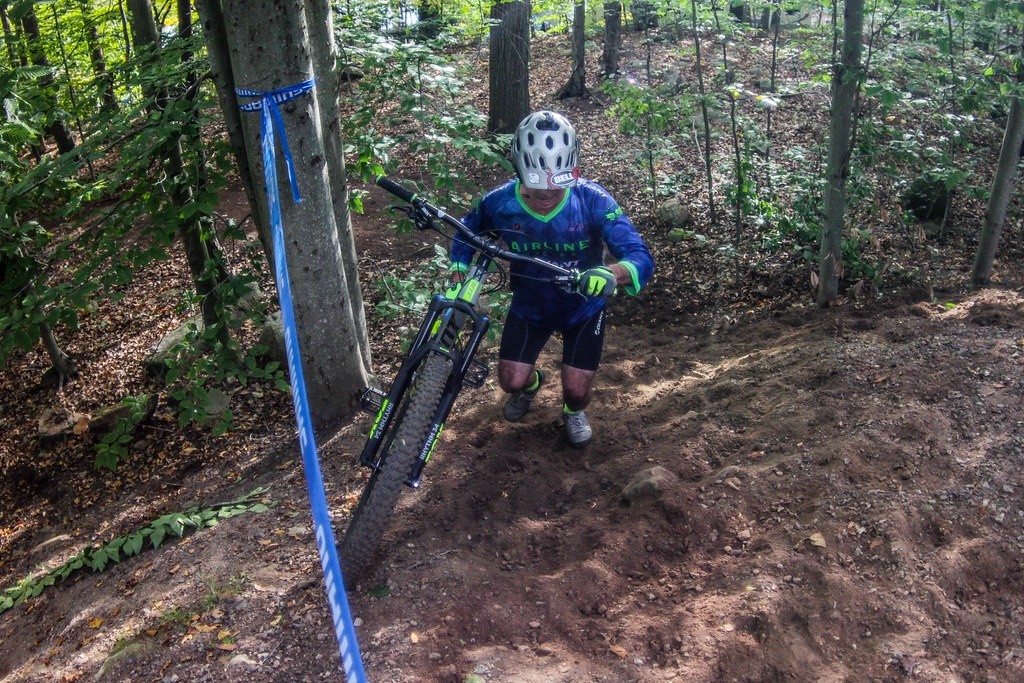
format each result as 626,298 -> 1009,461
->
576,266 -> 617,297
445,282 -> 462,300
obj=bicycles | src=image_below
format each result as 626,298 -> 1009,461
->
340,175 -> 617,592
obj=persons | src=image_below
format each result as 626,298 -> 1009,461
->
446,111 -> 655,448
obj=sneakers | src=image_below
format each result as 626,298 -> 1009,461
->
562,402 -> 593,448
503,367 -> 545,422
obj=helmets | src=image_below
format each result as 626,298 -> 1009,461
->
511,110 -> 581,190
